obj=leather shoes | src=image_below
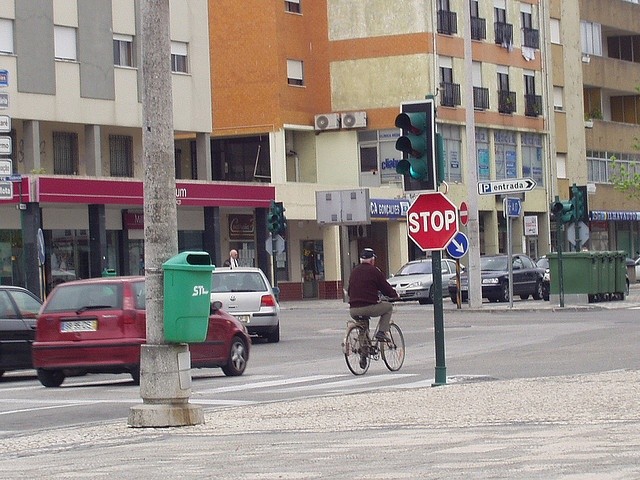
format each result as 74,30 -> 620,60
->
375,331 -> 392,340
359,358 -> 368,369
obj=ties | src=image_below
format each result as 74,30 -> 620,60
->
233,259 -> 238,268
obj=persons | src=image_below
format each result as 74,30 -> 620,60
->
223,249 -> 239,267
347,247 -> 400,369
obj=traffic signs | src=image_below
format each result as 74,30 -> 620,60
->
478,177 -> 537,195
1,69 -> 13,201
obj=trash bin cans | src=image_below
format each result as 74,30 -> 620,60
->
162,251 -> 215,343
101,269 -> 116,277
547,251 -> 599,303
615,250 -> 627,300
599,251 -> 609,302
607,251 -> 618,302
626,260 -> 636,284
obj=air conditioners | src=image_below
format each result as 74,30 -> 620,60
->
314,112 -> 339,131
341,112 -> 366,128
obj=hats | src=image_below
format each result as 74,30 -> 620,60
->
359,248 -> 378,259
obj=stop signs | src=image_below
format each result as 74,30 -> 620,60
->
406,191 -> 459,251
459,202 -> 469,226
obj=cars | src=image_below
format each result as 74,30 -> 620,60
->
31,276 -> 251,388
0,285 -> 45,374
379,260 -> 465,304
209,267 -> 281,344
536,252 -> 550,270
450,254 -> 547,304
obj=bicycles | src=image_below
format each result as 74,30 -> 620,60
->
341,295 -> 406,376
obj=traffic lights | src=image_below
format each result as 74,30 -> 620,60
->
268,202 -> 286,233
395,99 -> 437,194
552,201 -> 575,224
